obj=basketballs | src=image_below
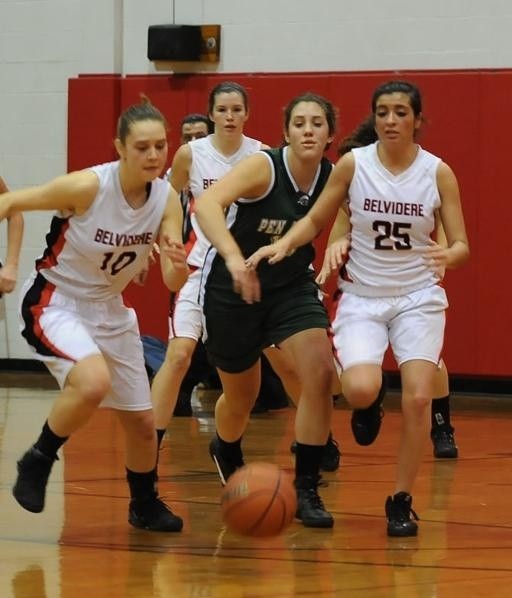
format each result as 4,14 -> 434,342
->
219,461 -> 298,541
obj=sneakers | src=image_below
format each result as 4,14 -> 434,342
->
293,473 -> 335,529
129,496 -> 183,533
430,424 -> 457,458
13,445 -> 59,513
317,434 -> 340,472
208,440 -> 245,485
385,491 -> 420,537
351,372 -> 386,446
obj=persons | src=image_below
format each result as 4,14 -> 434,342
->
152,81 -> 342,484
0,102 -> 189,532
245,81 -> 468,536
193,92 -> 337,528
0,177 -> 24,295
164,113 -> 267,417
